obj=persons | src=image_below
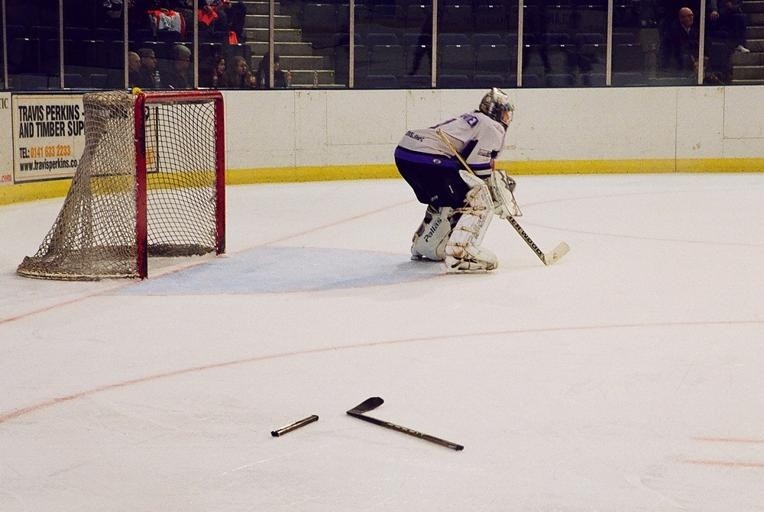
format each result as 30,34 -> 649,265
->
392,84 -> 522,275
85,1 -> 293,91
618,2 -> 752,86
383,1 -> 598,86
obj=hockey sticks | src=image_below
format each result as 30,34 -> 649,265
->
346,396 -> 465,451
438,128 -> 571,266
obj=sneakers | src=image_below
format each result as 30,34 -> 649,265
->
445,256 -> 493,270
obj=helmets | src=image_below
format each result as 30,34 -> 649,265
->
479,88 -> 513,128
